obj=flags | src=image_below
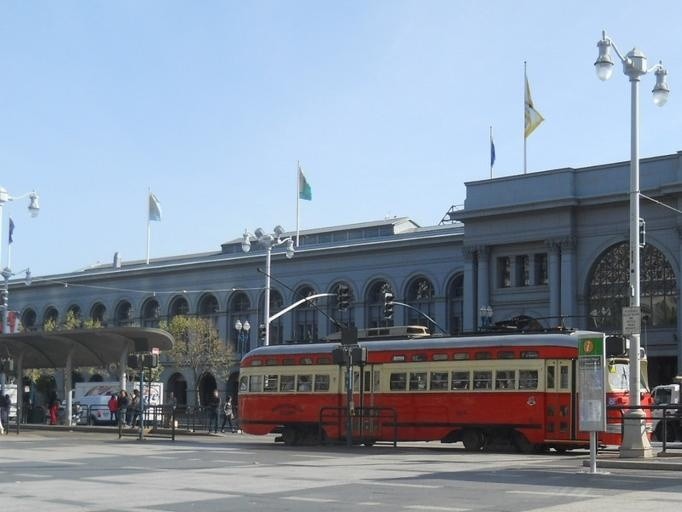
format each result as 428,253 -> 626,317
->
8,217 -> 15,244
149,192 -> 161,221
525,76 -> 545,139
298,169 -> 312,201
490,139 -> 496,167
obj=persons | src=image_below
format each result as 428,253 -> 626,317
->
108,388 -> 146,428
5,394 -> 11,421
49,397 -> 60,426
164,391 -> 177,428
1,389 -> 6,420
208,388 -> 221,434
221,395 -> 239,434
71,400 -> 84,424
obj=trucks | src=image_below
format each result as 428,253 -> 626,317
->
1,384 -> 31,423
61,380 -> 164,425
648,382 -> 681,443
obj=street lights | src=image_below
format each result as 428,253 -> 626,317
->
239,224 -> 295,343
479,304 -> 493,329
234,320 -> 250,362
594,28 -> 669,457
1,185 -> 40,396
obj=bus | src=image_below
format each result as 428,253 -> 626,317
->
237,326 -> 652,455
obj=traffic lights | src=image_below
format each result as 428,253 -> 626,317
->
338,284 -> 350,311
384,292 -> 393,319
